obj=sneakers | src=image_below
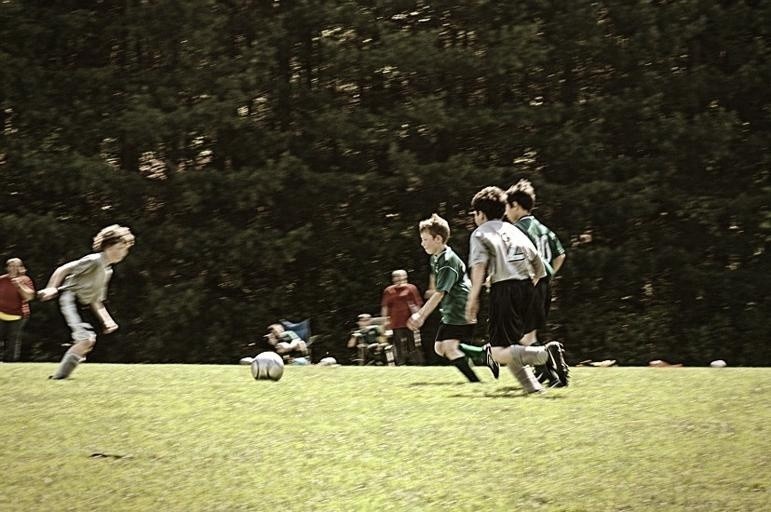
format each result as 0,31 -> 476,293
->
533,342 -> 569,387
481,342 -> 501,381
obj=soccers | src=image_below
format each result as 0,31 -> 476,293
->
251,351 -> 285,382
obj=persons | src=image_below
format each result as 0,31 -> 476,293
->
0,257 -> 37,362
262,322 -> 337,366
407,213 -> 499,382
466,186 -> 571,395
345,311 -> 385,350
37,224 -> 136,380
503,180 -> 567,385
380,270 -> 423,365
